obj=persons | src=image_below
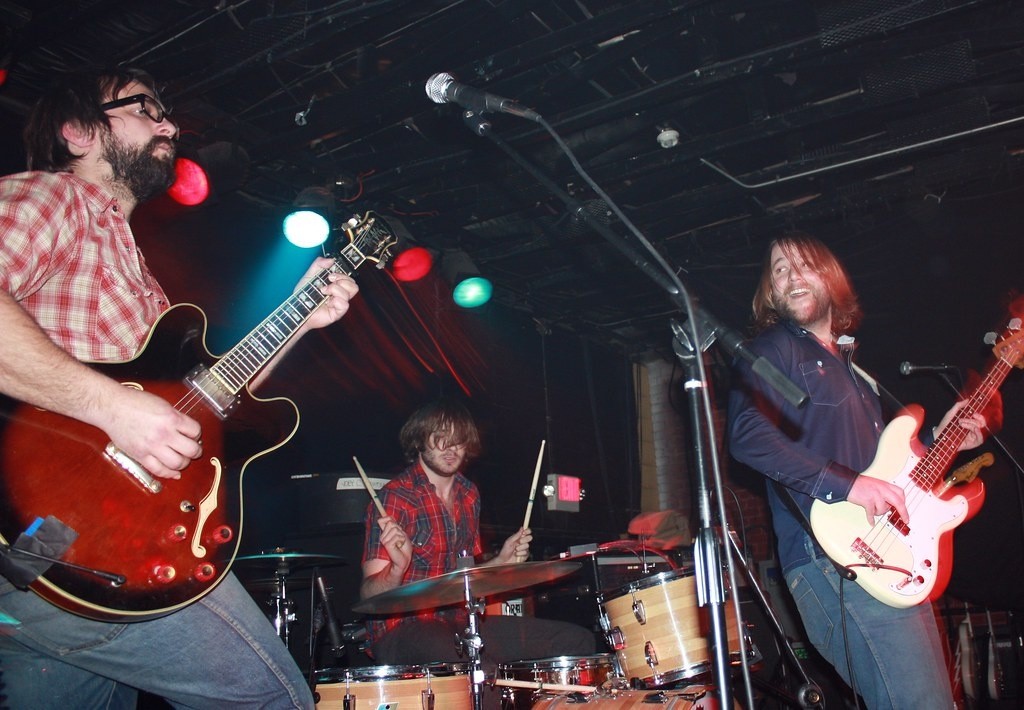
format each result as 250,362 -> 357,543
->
726,232 -> 985,710
361,400 -> 597,710
0,60 -> 358,710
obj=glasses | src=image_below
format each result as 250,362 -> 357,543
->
91,93 -> 181,143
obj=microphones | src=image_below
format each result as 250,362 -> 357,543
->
315,567 -> 345,658
425,72 -> 541,122
900,361 -> 956,375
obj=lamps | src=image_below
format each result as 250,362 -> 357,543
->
163,142 -> 252,211
441,249 -> 494,312
377,215 -> 432,281
280,185 -> 339,249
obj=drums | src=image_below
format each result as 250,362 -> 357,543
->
531,677 -> 743,710
497,652 -> 620,710
596,557 -> 764,690
311,660 -> 474,710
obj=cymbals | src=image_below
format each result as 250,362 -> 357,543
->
350,559 -> 585,616
234,546 -> 345,576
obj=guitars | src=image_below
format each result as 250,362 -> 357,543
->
808,317 -> 1024,611
0,209 -> 401,625
